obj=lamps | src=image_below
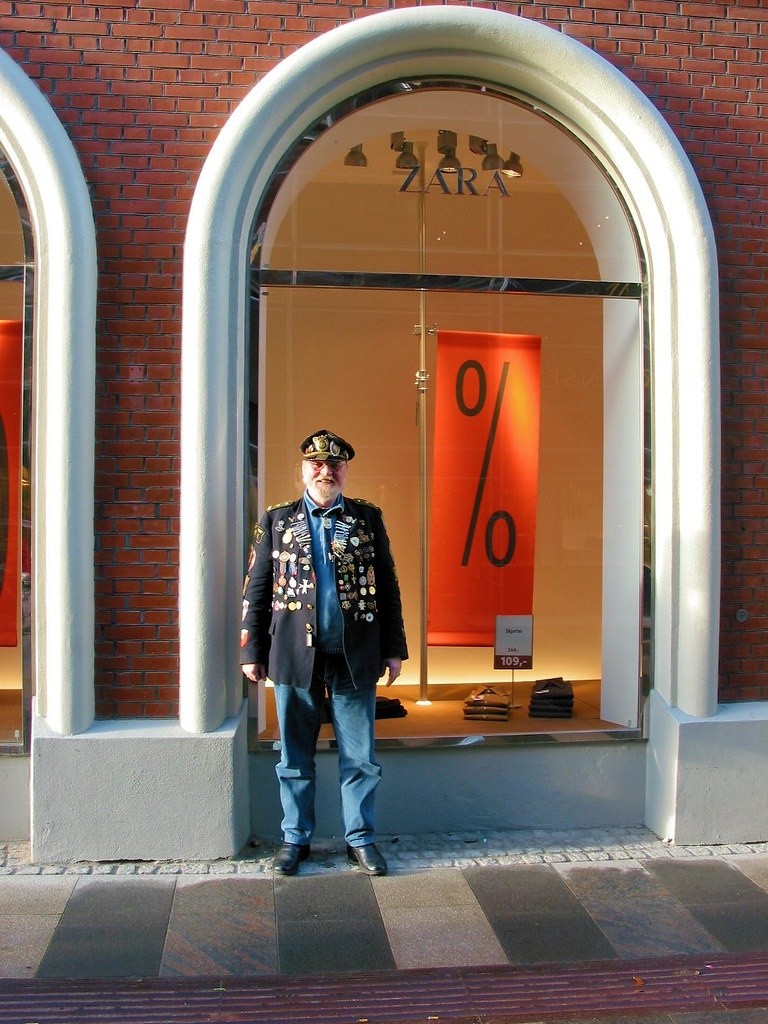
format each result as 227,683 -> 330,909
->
482,144 -> 504,170
500,152 -> 522,177
396,142 -> 418,170
344,143 -> 367,167
439,155 -> 461,174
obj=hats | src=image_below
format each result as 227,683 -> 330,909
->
300,428 -> 355,464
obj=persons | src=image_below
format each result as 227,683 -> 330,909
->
238,429 -> 410,876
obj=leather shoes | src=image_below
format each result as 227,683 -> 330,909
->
346,842 -> 388,876
272,842 -> 312,876
